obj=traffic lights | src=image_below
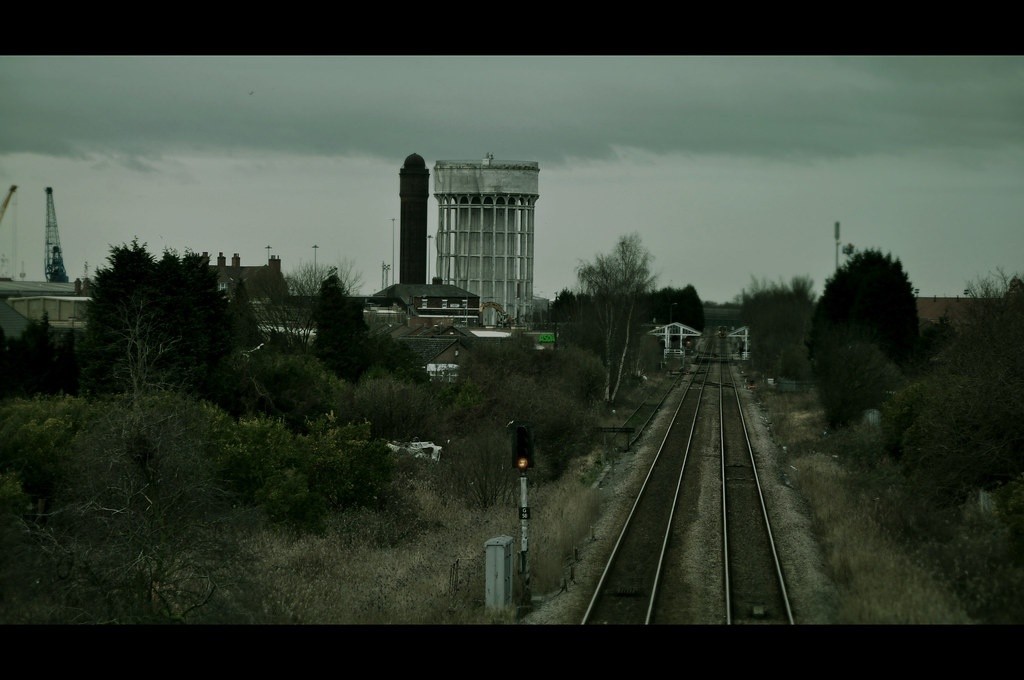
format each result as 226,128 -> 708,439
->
511,425 -> 534,470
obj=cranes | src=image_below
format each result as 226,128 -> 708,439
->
42,186 -> 75,285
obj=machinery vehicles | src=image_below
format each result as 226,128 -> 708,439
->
0,183 -> 27,284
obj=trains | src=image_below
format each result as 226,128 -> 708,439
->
718,325 -> 727,338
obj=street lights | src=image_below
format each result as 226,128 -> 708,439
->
387,218 -> 396,285
264,244 -> 272,264
312,243 -> 320,282
553,291 -> 560,349
427,235 -> 434,285
